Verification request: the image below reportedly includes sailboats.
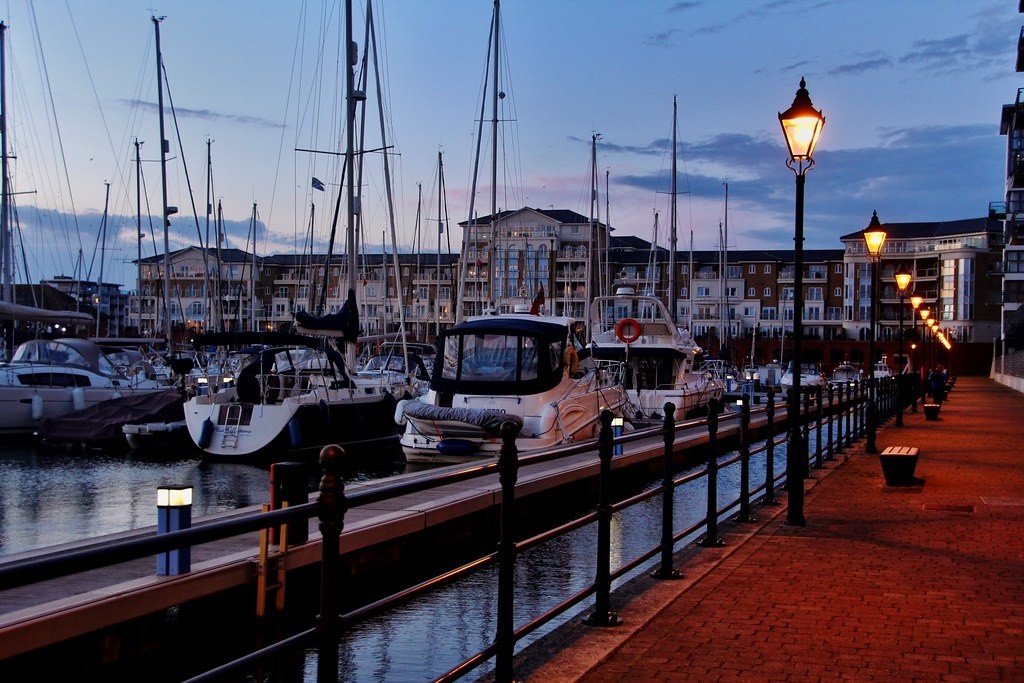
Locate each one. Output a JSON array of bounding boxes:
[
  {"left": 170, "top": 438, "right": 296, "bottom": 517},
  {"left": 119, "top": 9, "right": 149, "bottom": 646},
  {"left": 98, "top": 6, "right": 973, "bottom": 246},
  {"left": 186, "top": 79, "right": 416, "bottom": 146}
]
[{"left": 0, "top": 0, "right": 515, "bottom": 461}]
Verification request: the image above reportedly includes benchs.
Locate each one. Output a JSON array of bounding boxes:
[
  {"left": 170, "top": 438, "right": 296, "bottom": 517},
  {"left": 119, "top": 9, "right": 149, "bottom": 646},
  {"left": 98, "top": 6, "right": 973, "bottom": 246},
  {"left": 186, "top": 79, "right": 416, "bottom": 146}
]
[
  {"left": 923, "top": 404, "right": 940, "bottom": 420},
  {"left": 880, "top": 447, "right": 920, "bottom": 486},
  {"left": 943, "top": 376, "right": 956, "bottom": 401}
]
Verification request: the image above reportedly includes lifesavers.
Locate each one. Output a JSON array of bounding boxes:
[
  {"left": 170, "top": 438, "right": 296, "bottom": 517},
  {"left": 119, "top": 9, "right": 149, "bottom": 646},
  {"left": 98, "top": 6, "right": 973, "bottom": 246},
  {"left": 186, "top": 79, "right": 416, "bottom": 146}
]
[
  {"left": 563, "top": 346, "right": 579, "bottom": 374},
  {"left": 616, "top": 318, "right": 641, "bottom": 343}
]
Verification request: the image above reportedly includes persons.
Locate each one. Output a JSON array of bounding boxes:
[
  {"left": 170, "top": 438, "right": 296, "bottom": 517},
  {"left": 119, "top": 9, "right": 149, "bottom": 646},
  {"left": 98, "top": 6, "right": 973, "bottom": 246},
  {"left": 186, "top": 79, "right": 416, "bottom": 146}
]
[
  {"left": 765, "top": 378, "right": 769, "bottom": 386},
  {"left": 928, "top": 365, "right": 949, "bottom": 407}
]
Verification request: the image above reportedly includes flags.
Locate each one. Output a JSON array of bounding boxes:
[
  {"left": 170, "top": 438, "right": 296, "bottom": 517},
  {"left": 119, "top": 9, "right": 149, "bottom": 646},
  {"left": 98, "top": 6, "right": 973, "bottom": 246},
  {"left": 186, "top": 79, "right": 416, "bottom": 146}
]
[{"left": 530, "top": 288, "right": 544, "bottom": 316}]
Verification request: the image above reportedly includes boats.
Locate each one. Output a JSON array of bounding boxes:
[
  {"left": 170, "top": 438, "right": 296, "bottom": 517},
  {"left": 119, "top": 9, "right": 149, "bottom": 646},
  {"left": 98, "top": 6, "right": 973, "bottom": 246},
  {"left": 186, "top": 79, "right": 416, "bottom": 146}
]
[
  {"left": 587, "top": 293, "right": 723, "bottom": 422},
  {"left": 394, "top": 314, "right": 630, "bottom": 465},
  {"left": 868, "top": 363, "right": 893, "bottom": 387},
  {"left": 827, "top": 364, "right": 862, "bottom": 395},
  {"left": 779, "top": 357, "right": 827, "bottom": 398}
]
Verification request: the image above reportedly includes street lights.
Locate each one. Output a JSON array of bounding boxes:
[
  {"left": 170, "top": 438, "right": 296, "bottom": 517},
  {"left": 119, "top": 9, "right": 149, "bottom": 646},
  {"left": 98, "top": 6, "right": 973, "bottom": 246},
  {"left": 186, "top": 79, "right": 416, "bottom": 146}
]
[
  {"left": 893, "top": 262, "right": 912, "bottom": 428},
  {"left": 919, "top": 302, "right": 931, "bottom": 403},
  {"left": 862, "top": 210, "right": 888, "bottom": 453},
  {"left": 926, "top": 315, "right": 951, "bottom": 398},
  {"left": 909, "top": 288, "right": 923, "bottom": 412},
  {"left": 778, "top": 78, "right": 825, "bottom": 526}
]
